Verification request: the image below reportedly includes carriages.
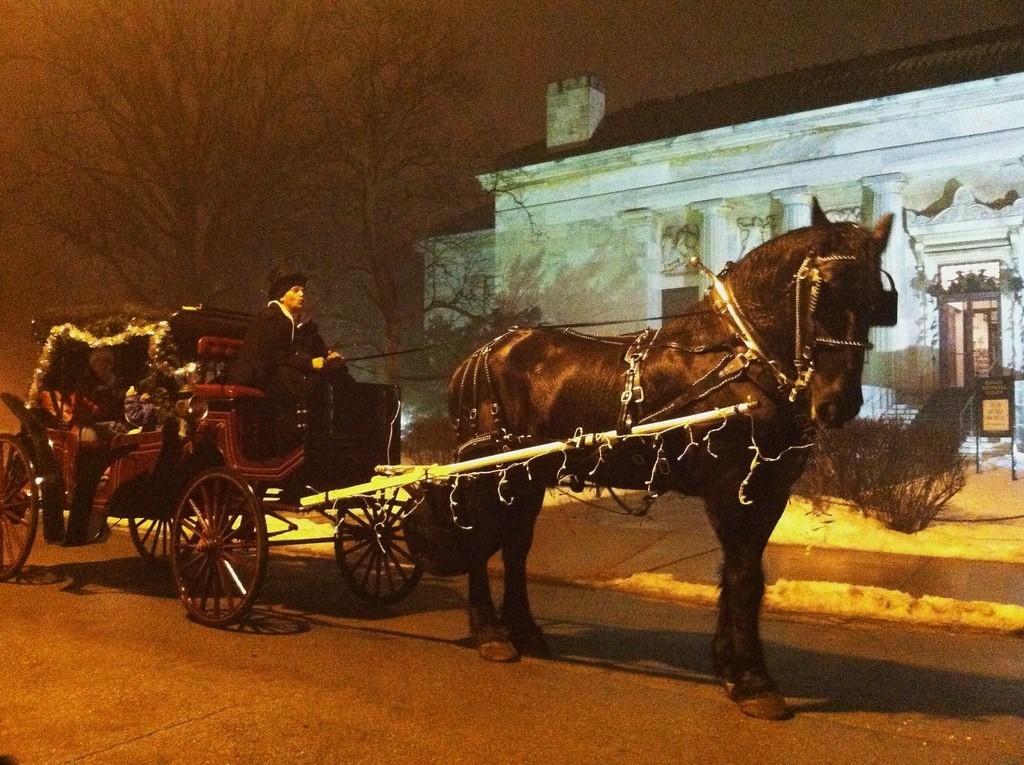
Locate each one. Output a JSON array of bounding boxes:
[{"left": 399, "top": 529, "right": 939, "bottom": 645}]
[{"left": 0, "top": 196, "right": 898, "bottom": 723}]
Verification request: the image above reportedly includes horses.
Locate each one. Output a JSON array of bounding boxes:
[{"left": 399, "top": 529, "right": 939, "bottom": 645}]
[{"left": 446, "top": 198, "right": 894, "bottom": 722}]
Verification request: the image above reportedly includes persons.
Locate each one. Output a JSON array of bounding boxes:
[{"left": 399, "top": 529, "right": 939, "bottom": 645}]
[
  {"left": 223, "top": 262, "right": 348, "bottom": 455},
  {"left": 58, "top": 347, "right": 164, "bottom": 430}
]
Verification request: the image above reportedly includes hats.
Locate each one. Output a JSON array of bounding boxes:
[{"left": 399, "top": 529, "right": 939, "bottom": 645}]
[{"left": 266, "top": 271, "right": 307, "bottom": 300}]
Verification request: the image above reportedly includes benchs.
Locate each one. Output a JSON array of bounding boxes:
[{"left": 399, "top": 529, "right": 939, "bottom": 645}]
[{"left": 193, "top": 335, "right": 269, "bottom": 409}]
